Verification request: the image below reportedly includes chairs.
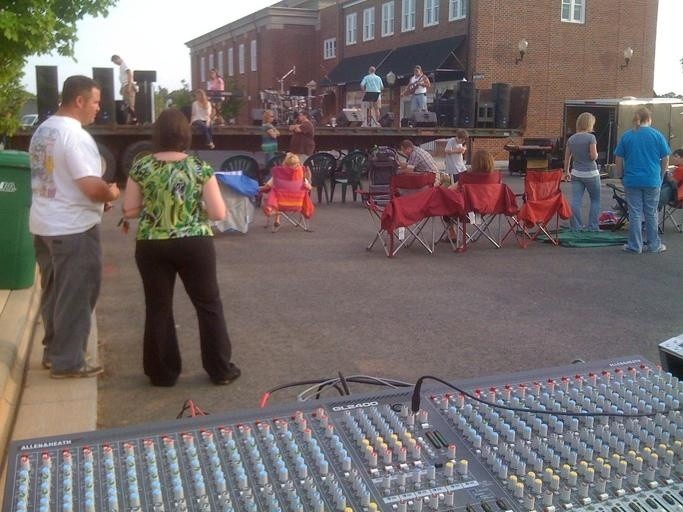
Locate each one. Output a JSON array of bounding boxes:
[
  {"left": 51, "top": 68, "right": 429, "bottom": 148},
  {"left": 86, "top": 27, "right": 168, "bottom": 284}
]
[
  {"left": 262, "top": 154, "right": 285, "bottom": 182},
  {"left": 604, "top": 174, "right": 677, "bottom": 234},
  {"left": 263, "top": 165, "right": 313, "bottom": 232},
  {"left": 657, "top": 199, "right": 682, "bottom": 232},
  {"left": 334, "top": 151, "right": 369, "bottom": 202},
  {"left": 219, "top": 155, "right": 259, "bottom": 179},
  {"left": 301, "top": 152, "right": 338, "bottom": 206},
  {"left": 354, "top": 169, "right": 572, "bottom": 258}
]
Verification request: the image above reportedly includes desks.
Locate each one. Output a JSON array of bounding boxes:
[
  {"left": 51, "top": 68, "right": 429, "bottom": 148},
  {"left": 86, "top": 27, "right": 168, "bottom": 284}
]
[
  {"left": 503, "top": 145, "right": 553, "bottom": 174},
  {"left": 207, "top": 171, "right": 259, "bottom": 234}
]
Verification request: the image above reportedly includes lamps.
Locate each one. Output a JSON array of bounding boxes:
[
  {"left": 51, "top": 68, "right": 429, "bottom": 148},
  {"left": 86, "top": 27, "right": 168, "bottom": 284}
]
[
  {"left": 619, "top": 46, "right": 633, "bottom": 70},
  {"left": 515, "top": 38, "right": 527, "bottom": 65}
]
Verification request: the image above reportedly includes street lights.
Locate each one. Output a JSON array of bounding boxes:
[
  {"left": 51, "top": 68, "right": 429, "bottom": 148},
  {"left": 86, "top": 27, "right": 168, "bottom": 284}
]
[
  {"left": 385, "top": 69, "right": 396, "bottom": 113},
  {"left": 309, "top": 79, "right": 316, "bottom": 108}
]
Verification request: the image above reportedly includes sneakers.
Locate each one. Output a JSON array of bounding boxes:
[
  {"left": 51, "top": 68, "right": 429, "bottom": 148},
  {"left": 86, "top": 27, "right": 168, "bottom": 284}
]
[
  {"left": 40, "top": 349, "right": 104, "bottom": 379},
  {"left": 210, "top": 366, "right": 241, "bottom": 385},
  {"left": 623, "top": 244, "right": 642, "bottom": 254},
  {"left": 647, "top": 244, "right": 667, "bottom": 253}
]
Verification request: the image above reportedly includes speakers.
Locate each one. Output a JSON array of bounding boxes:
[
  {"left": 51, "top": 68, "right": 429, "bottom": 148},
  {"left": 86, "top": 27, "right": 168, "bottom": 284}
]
[
  {"left": 337, "top": 108, "right": 365, "bottom": 126},
  {"left": 512, "top": 86, "right": 528, "bottom": 130},
  {"left": 94, "top": 67, "right": 114, "bottom": 126},
  {"left": 492, "top": 82, "right": 511, "bottom": 129},
  {"left": 252, "top": 109, "right": 276, "bottom": 125},
  {"left": 414, "top": 111, "right": 438, "bottom": 127},
  {"left": 457, "top": 81, "right": 476, "bottom": 128},
  {"left": 36, "top": 66, "right": 59, "bottom": 121}
]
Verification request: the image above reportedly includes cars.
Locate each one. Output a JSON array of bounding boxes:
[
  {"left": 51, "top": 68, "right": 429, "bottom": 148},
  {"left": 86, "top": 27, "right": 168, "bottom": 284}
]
[{"left": 18, "top": 112, "right": 39, "bottom": 128}]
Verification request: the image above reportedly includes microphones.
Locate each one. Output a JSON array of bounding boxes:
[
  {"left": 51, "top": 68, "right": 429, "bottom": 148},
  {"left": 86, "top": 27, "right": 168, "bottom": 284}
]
[{"left": 291, "top": 65, "right": 297, "bottom": 78}]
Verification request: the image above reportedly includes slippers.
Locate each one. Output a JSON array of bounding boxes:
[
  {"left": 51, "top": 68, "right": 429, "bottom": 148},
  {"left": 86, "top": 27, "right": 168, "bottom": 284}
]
[{"left": 440, "top": 235, "right": 457, "bottom": 243}]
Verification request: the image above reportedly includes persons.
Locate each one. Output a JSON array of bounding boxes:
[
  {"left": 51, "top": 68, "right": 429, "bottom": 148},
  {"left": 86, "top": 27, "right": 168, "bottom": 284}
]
[
  {"left": 124, "top": 107, "right": 242, "bottom": 388},
  {"left": 613, "top": 106, "right": 672, "bottom": 254},
  {"left": 398, "top": 139, "right": 438, "bottom": 188},
  {"left": 188, "top": 88, "right": 216, "bottom": 149},
  {"left": 443, "top": 127, "right": 470, "bottom": 223},
  {"left": 360, "top": 65, "right": 385, "bottom": 127},
  {"left": 27, "top": 75, "right": 122, "bottom": 379},
  {"left": 563, "top": 112, "right": 606, "bottom": 233},
  {"left": 255, "top": 109, "right": 317, "bottom": 227},
  {"left": 439, "top": 148, "right": 495, "bottom": 242},
  {"left": 111, "top": 54, "right": 139, "bottom": 125},
  {"left": 640, "top": 148, "right": 683, "bottom": 232},
  {"left": 404, "top": 64, "right": 431, "bottom": 128},
  {"left": 208, "top": 66, "right": 226, "bottom": 111}
]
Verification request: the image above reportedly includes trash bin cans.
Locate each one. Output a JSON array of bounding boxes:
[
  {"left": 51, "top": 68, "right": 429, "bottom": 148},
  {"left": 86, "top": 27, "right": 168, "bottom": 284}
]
[
  {"left": 608, "top": 164, "right": 616, "bottom": 176},
  {"left": 1, "top": 149, "right": 36, "bottom": 290}
]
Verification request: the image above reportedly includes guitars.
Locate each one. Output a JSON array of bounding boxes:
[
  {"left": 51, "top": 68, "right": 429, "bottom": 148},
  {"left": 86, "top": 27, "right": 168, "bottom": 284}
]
[{"left": 408, "top": 72, "right": 435, "bottom": 93}]
